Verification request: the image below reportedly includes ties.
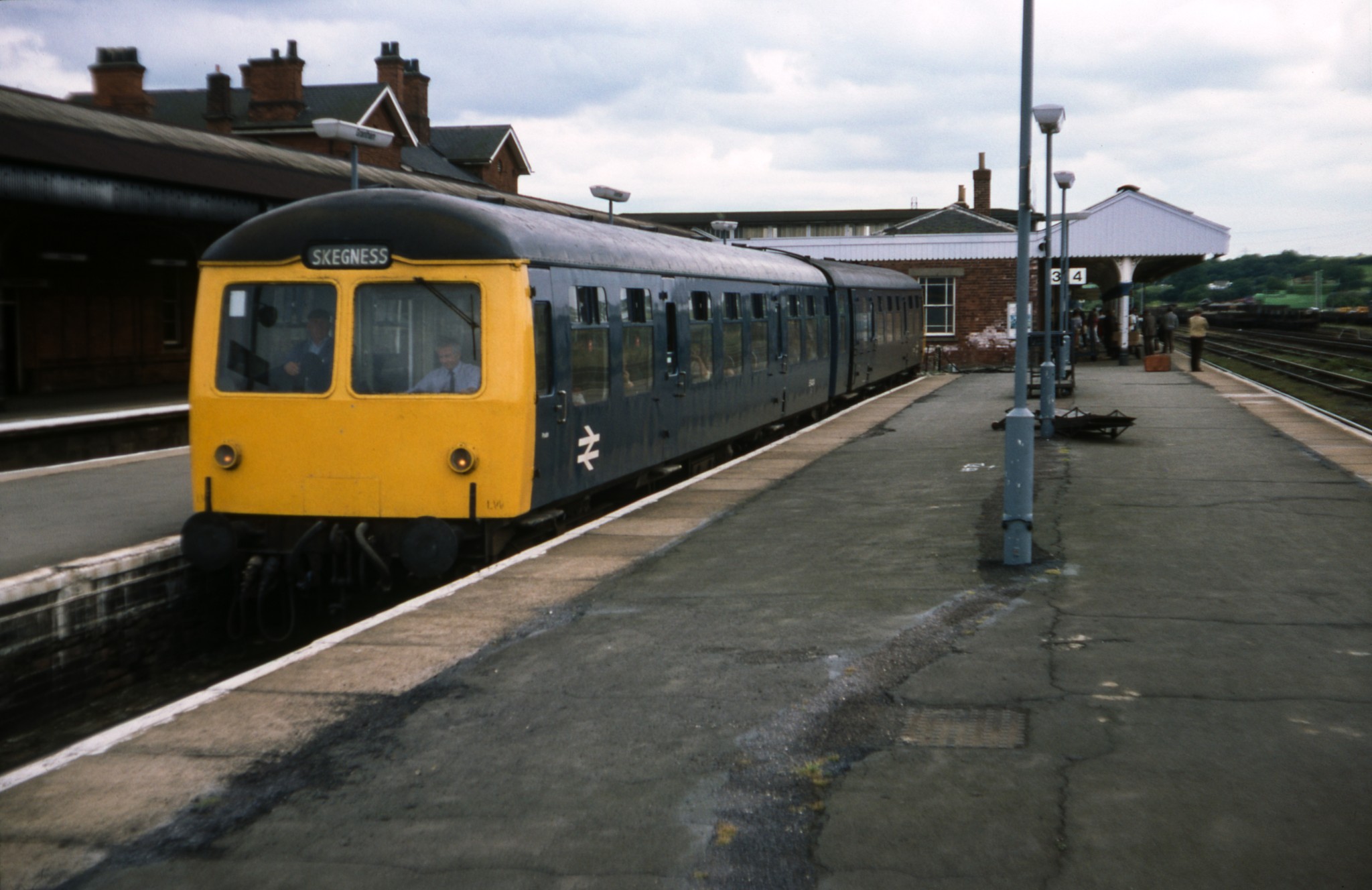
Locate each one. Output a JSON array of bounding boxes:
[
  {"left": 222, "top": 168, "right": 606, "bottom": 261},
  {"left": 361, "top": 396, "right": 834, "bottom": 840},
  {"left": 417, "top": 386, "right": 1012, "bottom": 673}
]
[{"left": 447, "top": 369, "right": 455, "bottom": 394}]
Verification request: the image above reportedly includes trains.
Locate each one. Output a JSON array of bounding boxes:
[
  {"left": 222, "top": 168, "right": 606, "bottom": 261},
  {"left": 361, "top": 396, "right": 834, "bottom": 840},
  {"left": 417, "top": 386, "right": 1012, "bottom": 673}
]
[{"left": 179, "top": 187, "right": 924, "bottom": 582}]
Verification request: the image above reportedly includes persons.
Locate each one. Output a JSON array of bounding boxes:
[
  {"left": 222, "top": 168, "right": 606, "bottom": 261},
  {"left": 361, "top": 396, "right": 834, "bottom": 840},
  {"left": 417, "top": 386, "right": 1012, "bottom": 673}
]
[
  {"left": 689, "top": 356, "right": 711, "bottom": 382},
  {"left": 572, "top": 385, "right": 586, "bottom": 407},
  {"left": 623, "top": 370, "right": 634, "bottom": 388},
  {"left": 1189, "top": 307, "right": 1209, "bottom": 372},
  {"left": 404, "top": 337, "right": 481, "bottom": 395},
  {"left": 271, "top": 308, "right": 334, "bottom": 393},
  {"left": 1070, "top": 303, "right": 1179, "bottom": 363}
]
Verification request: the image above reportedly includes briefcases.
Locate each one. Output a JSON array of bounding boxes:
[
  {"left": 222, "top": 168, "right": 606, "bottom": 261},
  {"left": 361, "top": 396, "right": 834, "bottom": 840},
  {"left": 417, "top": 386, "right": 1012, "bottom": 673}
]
[{"left": 1143, "top": 354, "right": 1171, "bottom": 372}]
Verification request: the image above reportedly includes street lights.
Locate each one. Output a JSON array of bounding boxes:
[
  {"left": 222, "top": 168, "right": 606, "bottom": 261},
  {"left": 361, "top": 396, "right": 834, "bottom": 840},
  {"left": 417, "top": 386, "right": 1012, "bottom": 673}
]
[
  {"left": 1032, "top": 100, "right": 1066, "bottom": 442},
  {"left": 311, "top": 119, "right": 394, "bottom": 192},
  {"left": 1053, "top": 169, "right": 1076, "bottom": 389},
  {"left": 710, "top": 218, "right": 737, "bottom": 240},
  {"left": 589, "top": 185, "right": 631, "bottom": 225}
]
